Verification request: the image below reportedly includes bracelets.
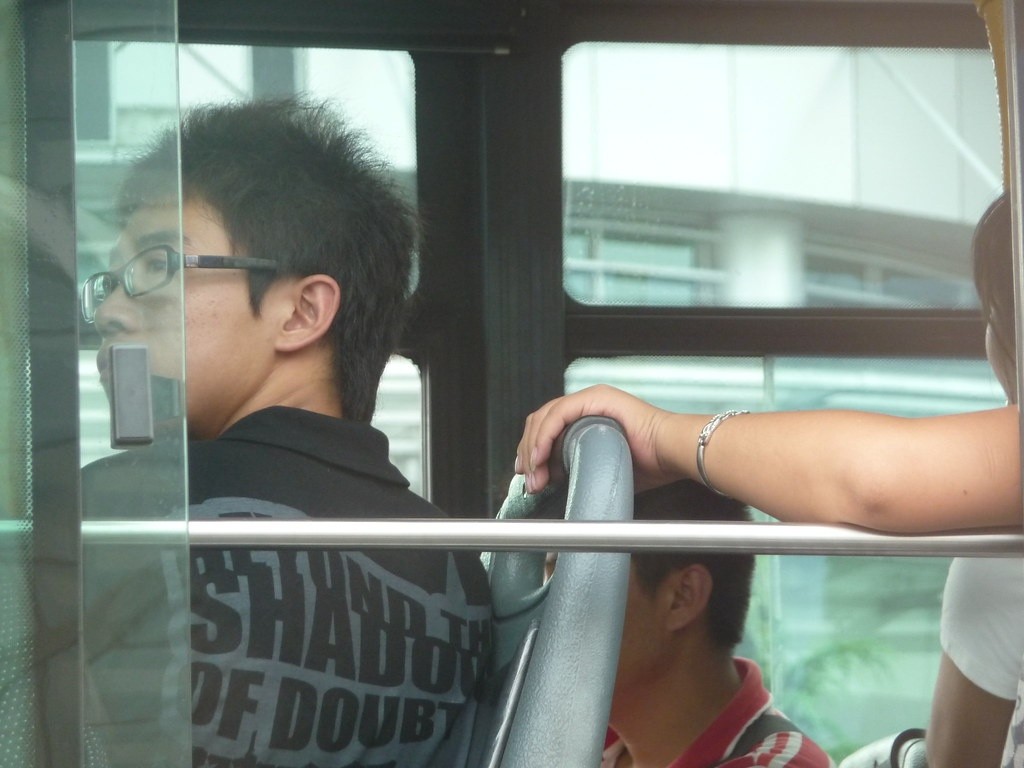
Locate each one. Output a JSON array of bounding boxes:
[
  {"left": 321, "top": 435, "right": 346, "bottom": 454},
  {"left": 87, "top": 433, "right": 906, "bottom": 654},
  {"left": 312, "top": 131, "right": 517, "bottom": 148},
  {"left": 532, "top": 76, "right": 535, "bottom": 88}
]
[{"left": 697, "top": 409, "right": 750, "bottom": 500}]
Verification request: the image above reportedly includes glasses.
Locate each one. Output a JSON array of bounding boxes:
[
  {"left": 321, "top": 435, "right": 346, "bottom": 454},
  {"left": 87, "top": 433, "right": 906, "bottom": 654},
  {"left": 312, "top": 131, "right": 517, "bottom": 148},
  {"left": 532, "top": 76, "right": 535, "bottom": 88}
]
[{"left": 81, "top": 243, "right": 287, "bottom": 323}]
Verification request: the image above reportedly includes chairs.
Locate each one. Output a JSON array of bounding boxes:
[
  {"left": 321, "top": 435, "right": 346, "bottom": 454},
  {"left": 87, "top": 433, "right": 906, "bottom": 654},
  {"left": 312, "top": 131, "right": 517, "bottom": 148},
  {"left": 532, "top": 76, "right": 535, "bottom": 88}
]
[{"left": 426, "top": 415, "right": 636, "bottom": 768}]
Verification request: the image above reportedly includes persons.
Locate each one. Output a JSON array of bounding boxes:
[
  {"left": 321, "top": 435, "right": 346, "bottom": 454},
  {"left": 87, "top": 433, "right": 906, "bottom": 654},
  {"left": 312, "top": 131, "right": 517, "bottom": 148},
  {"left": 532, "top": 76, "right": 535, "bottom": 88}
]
[
  {"left": 80, "top": 98, "right": 493, "bottom": 768},
  {"left": 514, "top": 189, "right": 1024, "bottom": 768}
]
